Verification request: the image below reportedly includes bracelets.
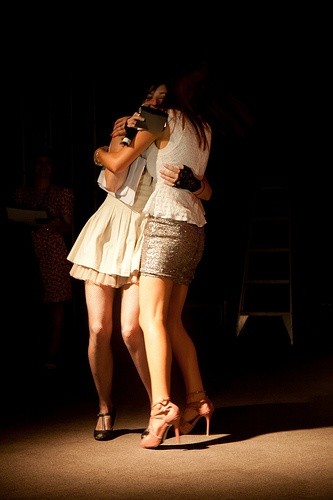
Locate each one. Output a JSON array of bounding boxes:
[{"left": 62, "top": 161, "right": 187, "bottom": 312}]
[
  {"left": 194, "top": 180, "right": 206, "bottom": 196},
  {"left": 93, "top": 150, "right": 103, "bottom": 166}
]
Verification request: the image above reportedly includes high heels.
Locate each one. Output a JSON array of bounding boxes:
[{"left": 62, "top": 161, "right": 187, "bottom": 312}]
[
  {"left": 93, "top": 411, "right": 115, "bottom": 440},
  {"left": 178, "top": 397, "right": 213, "bottom": 436},
  {"left": 140, "top": 398, "right": 181, "bottom": 449}
]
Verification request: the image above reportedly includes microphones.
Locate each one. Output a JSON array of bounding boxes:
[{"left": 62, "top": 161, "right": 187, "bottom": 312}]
[{"left": 122, "top": 102, "right": 148, "bottom": 145}]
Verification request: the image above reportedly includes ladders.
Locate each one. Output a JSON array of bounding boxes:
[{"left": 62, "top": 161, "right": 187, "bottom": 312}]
[{"left": 237, "top": 201, "right": 296, "bottom": 355}]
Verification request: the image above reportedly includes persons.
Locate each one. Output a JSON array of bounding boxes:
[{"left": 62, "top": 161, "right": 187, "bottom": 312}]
[
  {"left": 84, "top": 75, "right": 212, "bottom": 440},
  {"left": 15, "top": 151, "right": 74, "bottom": 370},
  {"left": 93, "top": 62, "right": 214, "bottom": 448}
]
